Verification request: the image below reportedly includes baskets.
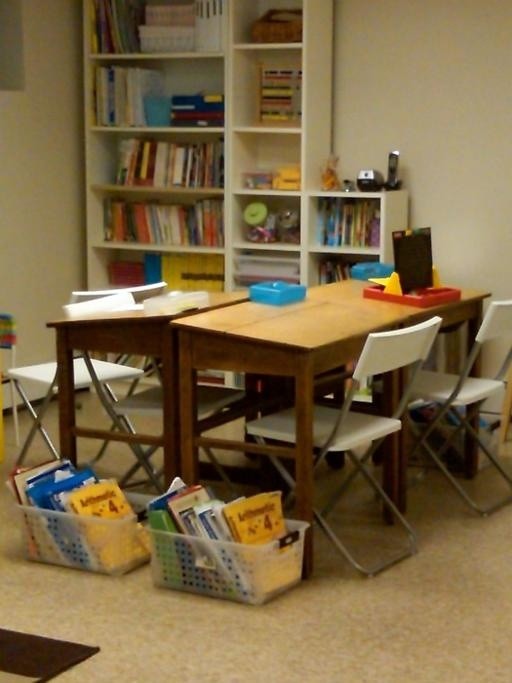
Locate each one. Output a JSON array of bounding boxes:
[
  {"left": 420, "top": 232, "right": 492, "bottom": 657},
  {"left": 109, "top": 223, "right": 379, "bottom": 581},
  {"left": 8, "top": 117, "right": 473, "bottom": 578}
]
[{"left": 14, "top": 492, "right": 162, "bottom": 578}]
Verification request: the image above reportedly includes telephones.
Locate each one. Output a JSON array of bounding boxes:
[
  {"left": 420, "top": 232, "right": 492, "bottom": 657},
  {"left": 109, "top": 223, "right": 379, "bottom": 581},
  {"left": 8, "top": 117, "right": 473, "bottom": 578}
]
[{"left": 383, "top": 151, "right": 403, "bottom": 191}]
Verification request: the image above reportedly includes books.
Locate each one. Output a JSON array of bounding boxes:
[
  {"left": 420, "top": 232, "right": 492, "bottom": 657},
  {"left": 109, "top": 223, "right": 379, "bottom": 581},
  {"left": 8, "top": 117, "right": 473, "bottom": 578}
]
[
  {"left": 91, "top": 350, "right": 226, "bottom": 387},
  {"left": 312, "top": 196, "right": 380, "bottom": 285},
  {"left": 344, "top": 362, "right": 494, "bottom": 469}
]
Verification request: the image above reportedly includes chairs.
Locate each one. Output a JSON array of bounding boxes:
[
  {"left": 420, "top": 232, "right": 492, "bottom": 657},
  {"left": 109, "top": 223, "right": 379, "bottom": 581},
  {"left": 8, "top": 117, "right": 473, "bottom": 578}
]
[{"left": 0, "top": 289, "right": 512, "bottom": 577}]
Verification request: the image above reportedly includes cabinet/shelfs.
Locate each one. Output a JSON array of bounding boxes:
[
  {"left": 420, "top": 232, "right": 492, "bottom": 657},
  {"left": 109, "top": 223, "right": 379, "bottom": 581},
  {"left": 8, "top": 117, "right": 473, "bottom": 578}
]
[{"left": 79, "top": 0, "right": 411, "bottom": 397}]
